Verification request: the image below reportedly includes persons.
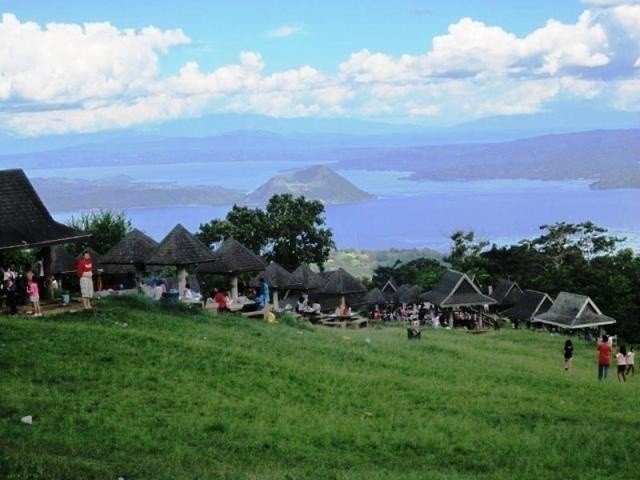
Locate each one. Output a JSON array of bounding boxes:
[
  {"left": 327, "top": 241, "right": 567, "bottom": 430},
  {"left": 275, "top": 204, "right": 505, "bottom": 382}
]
[
  {"left": 597, "top": 335, "right": 635, "bottom": 383},
  {"left": 137, "top": 277, "right": 270, "bottom": 313},
  {"left": 371, "top": 301, "right": 499, "bottom": 331},
  {"left": 296, "top": 296, "right": 351, "bottom": 321},
  {"left": 76, "top": 251, "right": 94, "bottom": 309},
  {"left": 563, "top": 339, "right": 574, "bottom": 371},
  {"left": 0, "top": 252, "right": 58, "bottom": 318}
]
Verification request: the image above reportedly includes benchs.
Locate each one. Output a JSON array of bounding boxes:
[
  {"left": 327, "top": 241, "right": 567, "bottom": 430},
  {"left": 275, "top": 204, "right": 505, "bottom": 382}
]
[{"left": 205, "top": 298, "right": 368, "bottom": 330}]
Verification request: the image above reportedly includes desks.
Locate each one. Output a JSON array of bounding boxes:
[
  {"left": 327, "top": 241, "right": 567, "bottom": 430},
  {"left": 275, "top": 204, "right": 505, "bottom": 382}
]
[{"left": 183, "top": 300, "right": 204, "bottom": 312}]
